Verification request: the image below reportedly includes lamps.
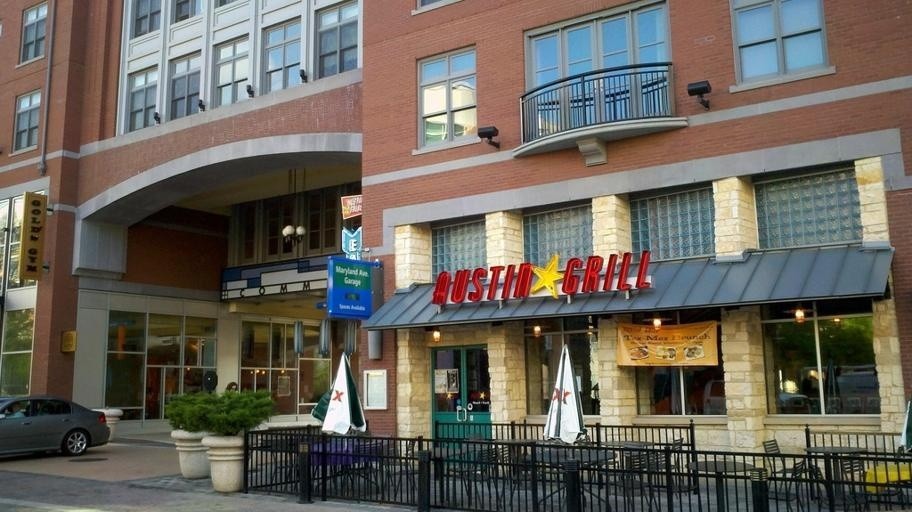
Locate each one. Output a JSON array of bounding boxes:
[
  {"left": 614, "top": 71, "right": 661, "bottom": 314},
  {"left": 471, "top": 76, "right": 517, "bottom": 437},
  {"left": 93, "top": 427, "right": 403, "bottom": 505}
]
[
  {"left": 246, "top": 85, "right": 253, "bottom": 98},
  {"left": 299, "top": 69, "right": 307, "bottom": 83},
  {"left": 475, "top": 126, "right": 500, "bottom": 150},
  {"left": 151, "top": 112, "right": 160, "bottom": 124},
  {"left": 197, "top": 100, "right": 204, "bottom": 112},
  {"left": 686, "top": 80, "right": 713, "bottom": 112},
  {"left": 280, "top": 168, "right": 308, "bottom": 247}
]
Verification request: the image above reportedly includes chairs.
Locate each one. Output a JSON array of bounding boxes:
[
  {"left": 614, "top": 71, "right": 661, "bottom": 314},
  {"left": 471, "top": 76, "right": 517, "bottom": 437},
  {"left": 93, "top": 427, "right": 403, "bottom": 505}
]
[
  {"left": 359, "top": 436, "right": 687, "bottom": 511},
  {"left": 840, "top": 455, "right": 904, "bottom": 511},
  {"left": 760, "top": 438, "right": 806, "bottom": 498}
]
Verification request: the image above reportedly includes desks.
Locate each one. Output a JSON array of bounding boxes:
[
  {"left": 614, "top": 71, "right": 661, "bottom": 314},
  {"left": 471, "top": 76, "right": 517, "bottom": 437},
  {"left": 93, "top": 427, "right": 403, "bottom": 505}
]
[
  {"left": 310, "top": 440, "right": 386, "bottom": 499},
  {"left": 801, "top": 445, "right": 868, "bottom": 508},
  {"left": 684, "top": 459, "right": 754, "bottom": 511}
]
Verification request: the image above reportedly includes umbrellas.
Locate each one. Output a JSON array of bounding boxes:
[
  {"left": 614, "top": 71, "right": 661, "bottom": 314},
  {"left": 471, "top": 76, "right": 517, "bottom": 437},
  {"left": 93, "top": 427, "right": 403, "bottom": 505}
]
[
  {"left": 899, "top": 399, "right": 912, "bottom": 449},
  {"left": 542, "top": 343, "right": 588, "bottom": 459},
  {"left": 308, "top": 351, "right": 368, "bottom": 435}
]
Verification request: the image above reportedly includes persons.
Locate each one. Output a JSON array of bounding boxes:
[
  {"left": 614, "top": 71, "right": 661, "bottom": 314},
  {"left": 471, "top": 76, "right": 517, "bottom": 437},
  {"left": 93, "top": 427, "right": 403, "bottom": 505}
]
[{"left": 6, "top": 401, "right": 26, "bottom": 419}]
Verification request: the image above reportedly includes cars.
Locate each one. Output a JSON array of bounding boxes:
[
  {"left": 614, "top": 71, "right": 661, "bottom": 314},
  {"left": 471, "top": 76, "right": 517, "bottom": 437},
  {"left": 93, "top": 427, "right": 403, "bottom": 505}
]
[{"left": 0, "top": 395, "right": 110, "bottom": 457}]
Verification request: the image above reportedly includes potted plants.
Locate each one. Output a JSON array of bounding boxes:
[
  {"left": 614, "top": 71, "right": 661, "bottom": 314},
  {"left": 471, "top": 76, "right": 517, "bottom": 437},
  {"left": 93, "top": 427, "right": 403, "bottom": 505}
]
[{"left": 169, "top": 387, "right": 276, "bottom": 492}]
